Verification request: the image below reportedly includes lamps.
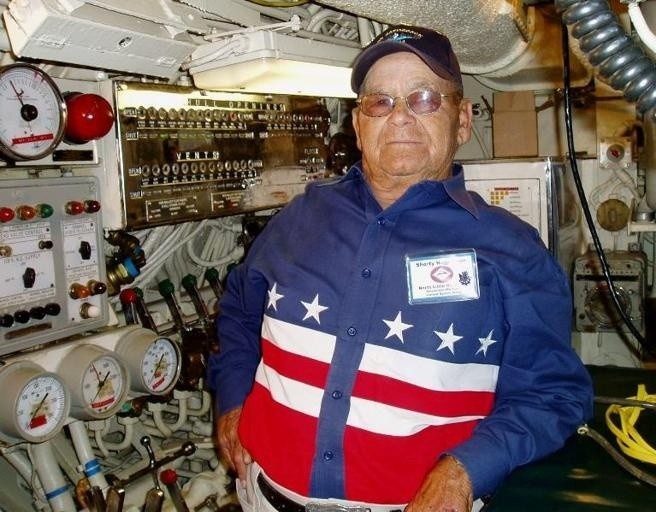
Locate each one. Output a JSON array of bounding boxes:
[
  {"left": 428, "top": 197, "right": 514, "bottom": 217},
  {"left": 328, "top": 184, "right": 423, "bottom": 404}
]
[{"left": 181, "top": 29, "right": 361, "bottom": 99}]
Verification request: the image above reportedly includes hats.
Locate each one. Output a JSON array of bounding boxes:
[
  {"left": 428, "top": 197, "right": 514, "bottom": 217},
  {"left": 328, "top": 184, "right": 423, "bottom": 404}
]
[{"left": 351, "top": 25, "right": 461, "bottom": 93}]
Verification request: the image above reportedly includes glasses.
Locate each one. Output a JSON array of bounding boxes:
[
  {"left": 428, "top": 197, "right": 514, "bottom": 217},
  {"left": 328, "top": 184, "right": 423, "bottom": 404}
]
[{"left": 356, "top": 89, "right": 456, "bottom": 117}]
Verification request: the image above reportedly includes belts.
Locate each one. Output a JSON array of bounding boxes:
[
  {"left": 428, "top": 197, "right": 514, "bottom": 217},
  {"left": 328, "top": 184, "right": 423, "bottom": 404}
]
[{"left": 257, "top": 470, "right": 380, "bottom": 512}]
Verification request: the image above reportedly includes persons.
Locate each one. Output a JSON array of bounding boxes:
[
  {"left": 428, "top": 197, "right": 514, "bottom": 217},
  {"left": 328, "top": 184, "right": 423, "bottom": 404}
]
[{"left": 202, "top": 23, "right": 595, "bottom": 512}]
